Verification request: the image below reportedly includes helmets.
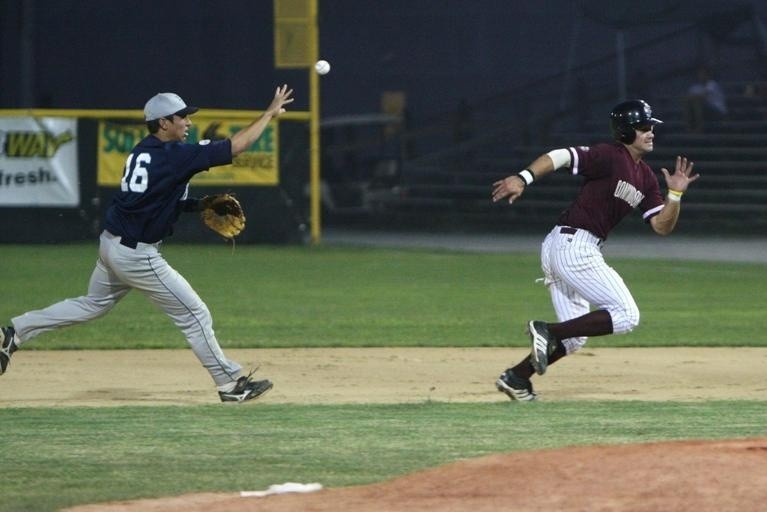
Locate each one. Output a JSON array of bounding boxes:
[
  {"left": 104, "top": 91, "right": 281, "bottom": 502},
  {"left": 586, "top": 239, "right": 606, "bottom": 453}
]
[{"left": 609, "top": 98, "right": 664, "bottom": 129}]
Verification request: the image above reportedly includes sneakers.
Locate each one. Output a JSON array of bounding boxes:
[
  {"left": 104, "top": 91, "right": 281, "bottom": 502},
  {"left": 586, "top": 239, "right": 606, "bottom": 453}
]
[
  {"left": 493, "top": 368, "right": 536, "bottom": 401},
  {"left": 526, "top": 318, "right": 558, "bottom": 376},
  {"left": 0, "top": 326, "right": 19, "bottom": 376},
  {"left": 216, "top": 374, "right": 273, "bottom": 403}
]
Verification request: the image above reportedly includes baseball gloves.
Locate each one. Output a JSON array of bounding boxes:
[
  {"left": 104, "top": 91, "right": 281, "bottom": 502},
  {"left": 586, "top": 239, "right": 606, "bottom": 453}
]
[{"left": 199, "top": 192, "right": 248, "bottom": 239}]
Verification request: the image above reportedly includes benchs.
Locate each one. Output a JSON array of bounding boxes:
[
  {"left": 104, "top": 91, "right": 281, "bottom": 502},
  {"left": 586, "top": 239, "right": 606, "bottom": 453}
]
[{"left": 394, "top": 35, "right": 767, "bottom": 237}]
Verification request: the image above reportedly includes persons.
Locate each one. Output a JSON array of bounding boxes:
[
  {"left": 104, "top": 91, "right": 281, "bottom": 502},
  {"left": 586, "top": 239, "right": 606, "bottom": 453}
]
[
  {"left": 0, "top": 82, "right": 298, "bottom": 402},
  {"left": 487, "top": 97, "right": 703, "bottom": 402}
]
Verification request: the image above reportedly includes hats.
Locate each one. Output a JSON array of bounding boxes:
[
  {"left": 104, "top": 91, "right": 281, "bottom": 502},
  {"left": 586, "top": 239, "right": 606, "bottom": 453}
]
[{"left": 143, "top": 92, "right": 198, "bottom": 123}]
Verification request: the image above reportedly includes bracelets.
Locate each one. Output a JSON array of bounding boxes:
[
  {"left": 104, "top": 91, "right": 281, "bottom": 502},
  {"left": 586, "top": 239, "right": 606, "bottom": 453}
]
[
  {"left": 516, "top": 169, "right": 535, "bottom": 188},
  {"left": 667, "top": 189, "right": 682, "bottom": 196},
  {"left": 667, "top": 193, "right": 682, "bottom": 202}
]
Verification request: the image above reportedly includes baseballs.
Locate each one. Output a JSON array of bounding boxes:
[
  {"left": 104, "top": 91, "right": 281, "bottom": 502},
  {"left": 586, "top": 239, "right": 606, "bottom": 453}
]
[{"left": 316, "top": 60, "right": 331, "bottom": 75}]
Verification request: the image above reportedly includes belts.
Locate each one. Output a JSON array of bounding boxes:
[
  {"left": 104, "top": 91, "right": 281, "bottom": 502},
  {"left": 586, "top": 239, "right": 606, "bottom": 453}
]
[
  {"left": 553, "top": 225, "right": 601, "bottom": 246},
  {"left": 101, "top": 229, "right": 153, "bottom": 250}
]
